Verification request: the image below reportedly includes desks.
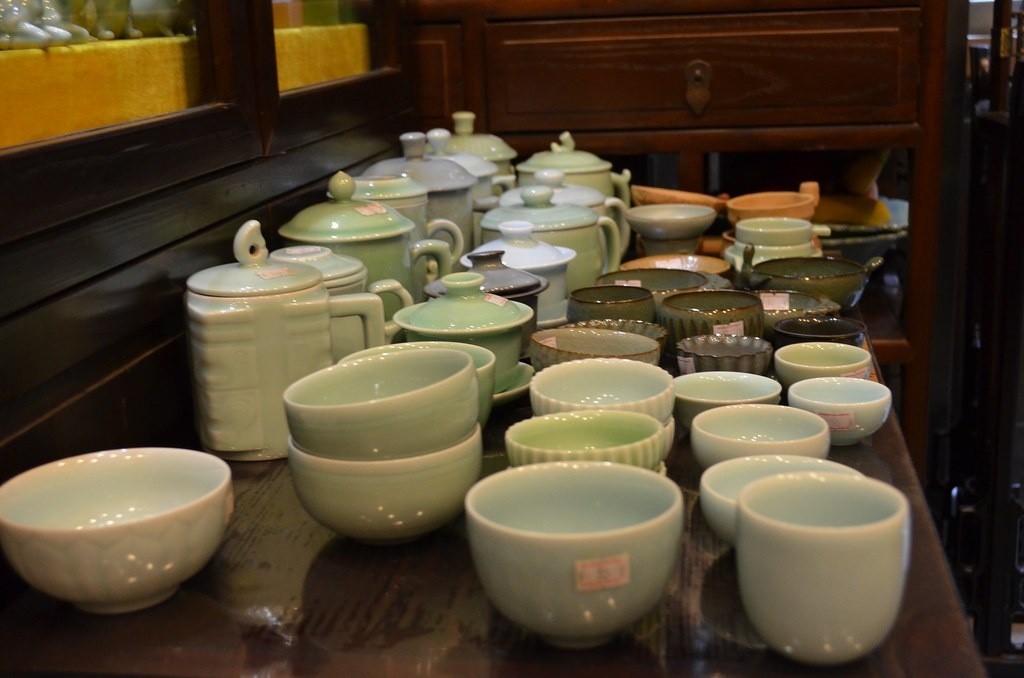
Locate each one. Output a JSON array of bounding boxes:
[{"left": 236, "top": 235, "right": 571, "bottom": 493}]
[{"left": 0, "top": 306, "right": 988, "bottom": 678}]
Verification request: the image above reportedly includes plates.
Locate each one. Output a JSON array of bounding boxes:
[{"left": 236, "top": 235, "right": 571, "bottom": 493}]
[
  {"left": 538, "top": 307, "right": 572, "bottom": 329},
  {"left": 492, "top": 359, "right": 536, "bottom": 402}
]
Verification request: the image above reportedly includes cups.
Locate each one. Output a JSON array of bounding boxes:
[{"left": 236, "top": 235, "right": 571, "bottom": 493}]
[
  {"left": 737, "top": 471, "right": 911, "bottom": 662},
  {"left": 182, "top": 110, "right": 631, "bottom": 461}
]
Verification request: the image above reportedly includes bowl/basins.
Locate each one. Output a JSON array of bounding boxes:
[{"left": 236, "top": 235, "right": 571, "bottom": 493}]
[
  {"left": 465, "top": 319, "right": 685, "bottom": 648},
  {"left": 772, "top": 341, "right": 891, "bottom": 447},
  {"left": 283, "top": 341, "right": 496, "bottom": 539},
  {"left": 660, "top": 288, "right": 865, "bottom": 547},
  {"left": 724, "top": 182, "right": 868, "bottom": 348},
  {"left": 0, "top": 448, "right": 233, "bottom": 614},
  {"left": 591, "top": 183, "right": 733, "bottom": 322}
]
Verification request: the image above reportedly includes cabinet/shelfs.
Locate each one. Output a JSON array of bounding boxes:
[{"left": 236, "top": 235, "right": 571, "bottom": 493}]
[{"left": 410, "top": 0, "right": 947, "bottom": 482}]
